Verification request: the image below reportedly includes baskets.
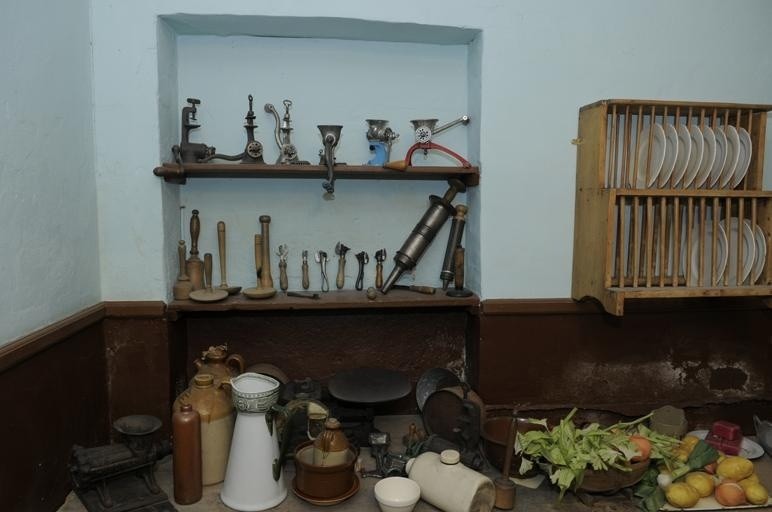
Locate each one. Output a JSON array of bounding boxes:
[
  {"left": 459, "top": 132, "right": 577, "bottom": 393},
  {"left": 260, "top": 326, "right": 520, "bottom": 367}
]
[{"left": 579, "top": 460, "right": 651, "bottom": 492}]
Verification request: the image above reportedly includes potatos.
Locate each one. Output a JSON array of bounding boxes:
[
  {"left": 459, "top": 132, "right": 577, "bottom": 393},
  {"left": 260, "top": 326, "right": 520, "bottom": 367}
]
[{"left": 663, "top": 436, "right": 768, "bottom": 508}]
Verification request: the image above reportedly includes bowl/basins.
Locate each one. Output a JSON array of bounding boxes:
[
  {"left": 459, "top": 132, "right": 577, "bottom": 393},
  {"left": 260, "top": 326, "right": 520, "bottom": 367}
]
[
  {"left": 415, "top": 365, "right": 468, "bottom": 415},
  {"left": 484, "top": 406, "right": 658, "bottom": 495},
  {"left": 290, "top": 440, "right": 363, "bottom": 501},
  {"left": 372, "top": 476, "right": 422, "bottom": 509}
]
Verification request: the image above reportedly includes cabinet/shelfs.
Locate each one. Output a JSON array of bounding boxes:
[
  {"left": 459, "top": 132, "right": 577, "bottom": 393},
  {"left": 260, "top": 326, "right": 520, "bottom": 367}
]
[
  {"left": 163, "top": 162, "right": 481, "bottom": 313},
  {"left": 571, "top": 98, "right": 772, "bottom": 318}
]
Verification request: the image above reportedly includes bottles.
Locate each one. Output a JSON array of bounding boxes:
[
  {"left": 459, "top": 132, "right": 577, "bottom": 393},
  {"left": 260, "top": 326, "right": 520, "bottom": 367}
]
[
  {"left": 313, "top": 418, "right": 350, "bottom": 467},
  {"left": 172, "top": 403, "right": 203, "bottom": 504}
]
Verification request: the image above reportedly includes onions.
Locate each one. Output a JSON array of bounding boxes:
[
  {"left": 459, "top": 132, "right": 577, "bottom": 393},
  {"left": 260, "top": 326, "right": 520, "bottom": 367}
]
[{"left": 628, "top": 436, "right": 651, "bottom": 462}]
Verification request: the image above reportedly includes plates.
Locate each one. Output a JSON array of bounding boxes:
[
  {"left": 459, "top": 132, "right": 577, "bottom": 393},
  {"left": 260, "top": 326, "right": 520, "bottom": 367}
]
[
  {"left": 685, "top": 429, "right": 765, "bottom": 460},
  {"left": 633, "top": 118, "right": 767, "bottom": 289}
]
